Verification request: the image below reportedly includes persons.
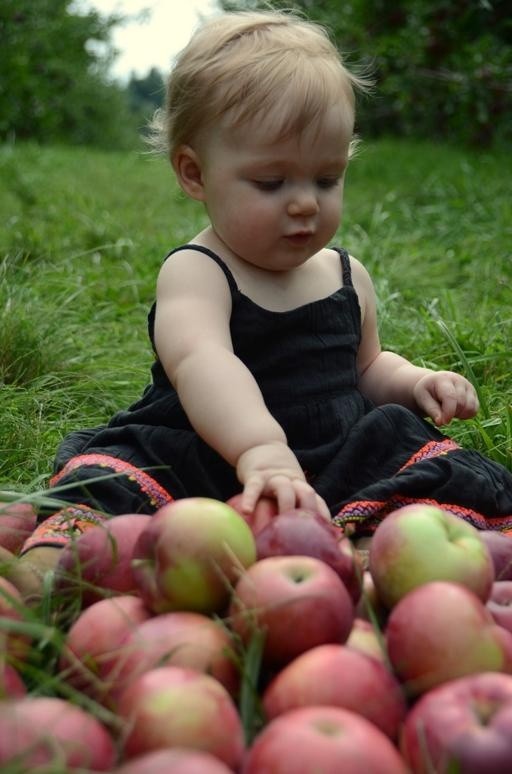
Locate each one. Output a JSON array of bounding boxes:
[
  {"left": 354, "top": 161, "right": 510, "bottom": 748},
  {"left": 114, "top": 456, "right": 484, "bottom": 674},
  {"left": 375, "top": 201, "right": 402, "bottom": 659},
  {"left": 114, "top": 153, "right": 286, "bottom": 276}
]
[{"left": 17, "top": 7, "right": 512, "bottom": 560}]
